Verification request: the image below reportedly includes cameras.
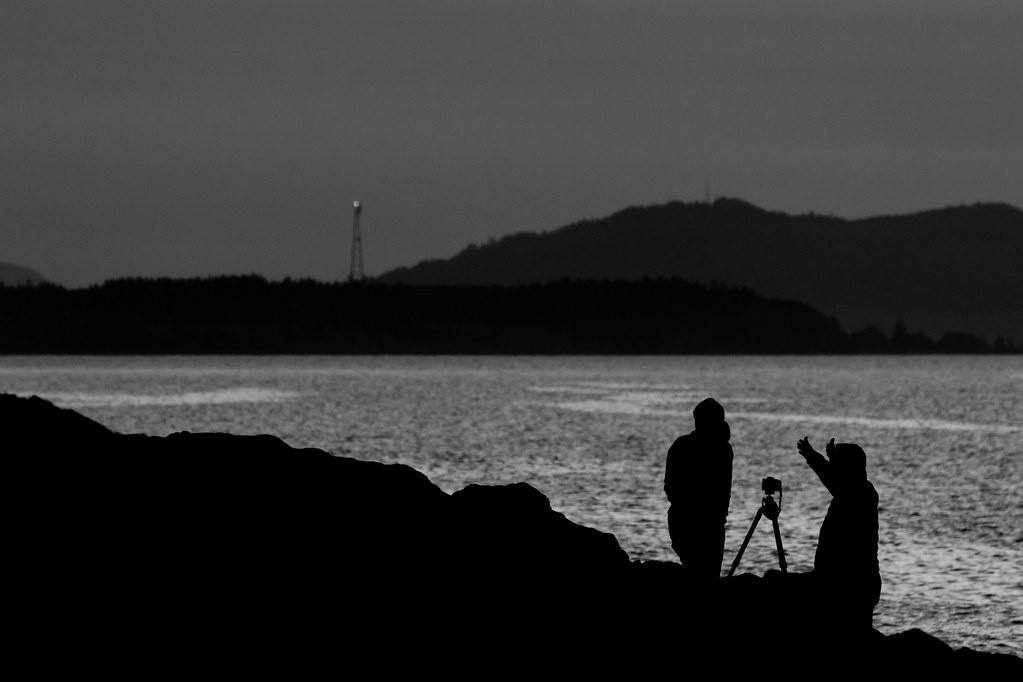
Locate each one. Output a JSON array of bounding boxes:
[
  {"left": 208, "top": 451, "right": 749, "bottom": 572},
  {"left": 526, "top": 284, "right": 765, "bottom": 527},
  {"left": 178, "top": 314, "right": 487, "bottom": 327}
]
[{"left": 762, "top": 476, "right": 782, "bottom": 495}]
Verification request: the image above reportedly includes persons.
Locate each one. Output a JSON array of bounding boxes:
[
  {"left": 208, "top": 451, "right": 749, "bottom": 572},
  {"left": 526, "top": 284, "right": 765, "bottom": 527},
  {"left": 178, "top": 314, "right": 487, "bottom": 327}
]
[
  {"left": 796, "top": 432, "right": 883, "bottom": 623},
  {"left": 661, "top": 396, "right": 733, "bottom": 582}
]
